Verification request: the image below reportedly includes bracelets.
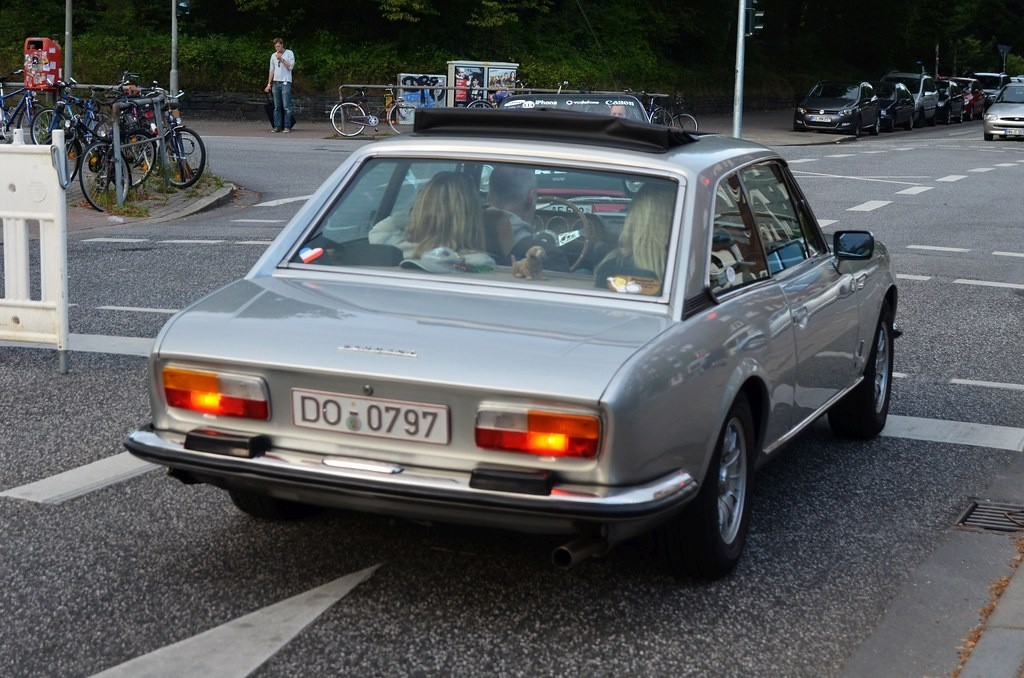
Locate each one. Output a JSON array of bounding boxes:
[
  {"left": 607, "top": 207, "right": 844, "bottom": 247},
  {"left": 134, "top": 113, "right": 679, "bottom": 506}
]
[
  {"left": 267, "top": 84, "right": 272, "bottom": 86},
  {"left": 281, "top": 59, "right": 285, "bottom": 63}
]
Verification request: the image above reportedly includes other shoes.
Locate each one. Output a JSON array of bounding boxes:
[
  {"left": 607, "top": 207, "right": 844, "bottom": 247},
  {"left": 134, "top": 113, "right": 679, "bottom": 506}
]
[
  {"left": 282, "top": 127, "right": 291, "bottom": 133},
  {"left": 271, "top": 127, "right": 280, "bottom": 133}
]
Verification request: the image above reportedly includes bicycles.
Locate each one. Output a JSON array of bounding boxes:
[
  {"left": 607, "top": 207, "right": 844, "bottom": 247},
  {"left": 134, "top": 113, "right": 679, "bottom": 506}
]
[
  {"left": 329, "top": 82, "right": 416, "bottom": 137},
  {"left": 0, "top": 69, "right": 51, "bottom": 145},
  {"left": 466, "top": 78, "right": 532, "bottom": 108},
  {"left": 29, "top": 70, "right": 206, "bottom": 214},
  {"left": 625, "top": 88, "right": 699, "bottom": 132}
]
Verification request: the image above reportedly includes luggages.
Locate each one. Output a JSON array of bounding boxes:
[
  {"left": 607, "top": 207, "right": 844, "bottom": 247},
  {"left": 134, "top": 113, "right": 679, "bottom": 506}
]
[{"left": 264, "top": 91, "right": 296, "bottom": 130}]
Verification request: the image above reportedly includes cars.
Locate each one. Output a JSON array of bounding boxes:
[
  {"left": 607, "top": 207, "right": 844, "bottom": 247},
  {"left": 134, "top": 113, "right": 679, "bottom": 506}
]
[
  {"left": 941, "top": 75, "right": 987, "bottom": 122},
  {"left": 930, "top": 79, "right": 965, "bottom": 125},
  {"left": 862, "top": 80, "right": 916, "bottom": 135},
  {"left": 983, "top": 82, "right": 1024, "bottom": 141},
  {"left": 495, "top": 90, "right": 677, "bottom": 126},
  {"left": 793, "top": 79, "right": 883, "bottom": 138}
]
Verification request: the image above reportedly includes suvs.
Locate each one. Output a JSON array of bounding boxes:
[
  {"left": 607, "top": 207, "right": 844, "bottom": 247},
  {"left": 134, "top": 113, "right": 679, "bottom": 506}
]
[
  {"left": 968, "top": 72, "right": 1024, "bottom": 112},
  {"left": 880, "top": 70, "right": 940, "bottom": 129}
]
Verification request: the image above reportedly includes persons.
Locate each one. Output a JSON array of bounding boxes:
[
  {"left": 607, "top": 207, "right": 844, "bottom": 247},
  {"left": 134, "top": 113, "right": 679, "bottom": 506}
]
[
  {"left": 486, "top": 164, "right": 570, "bottom": 273},
  {"left": 265, "top": 38, "right": 295, "bottom": 133},
  {"left": 595, "top": 181, "right": 678, "bottom": 274},
  {"left": 611, "top": 105, "right": 630, "bottom": 119},
  {"left": 368, "top": 171, "right": 496, "bottom": 264},
  {"left": 489, "top": 72, "right": 516, "bottom": 93},
  {"left": 470, "top": 77, "right": 482, "bottom": 100}
]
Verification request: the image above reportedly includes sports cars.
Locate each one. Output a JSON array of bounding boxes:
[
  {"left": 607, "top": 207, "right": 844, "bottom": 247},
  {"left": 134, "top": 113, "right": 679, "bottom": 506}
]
[{"left": 122, "top": 103, "right": 906, "bottom": 586}]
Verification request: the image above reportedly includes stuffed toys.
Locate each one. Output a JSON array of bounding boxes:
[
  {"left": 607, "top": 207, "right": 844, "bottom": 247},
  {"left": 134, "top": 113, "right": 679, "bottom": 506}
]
[{"left": 511, "top": 246, "right": 547, "bottom": 280}]
